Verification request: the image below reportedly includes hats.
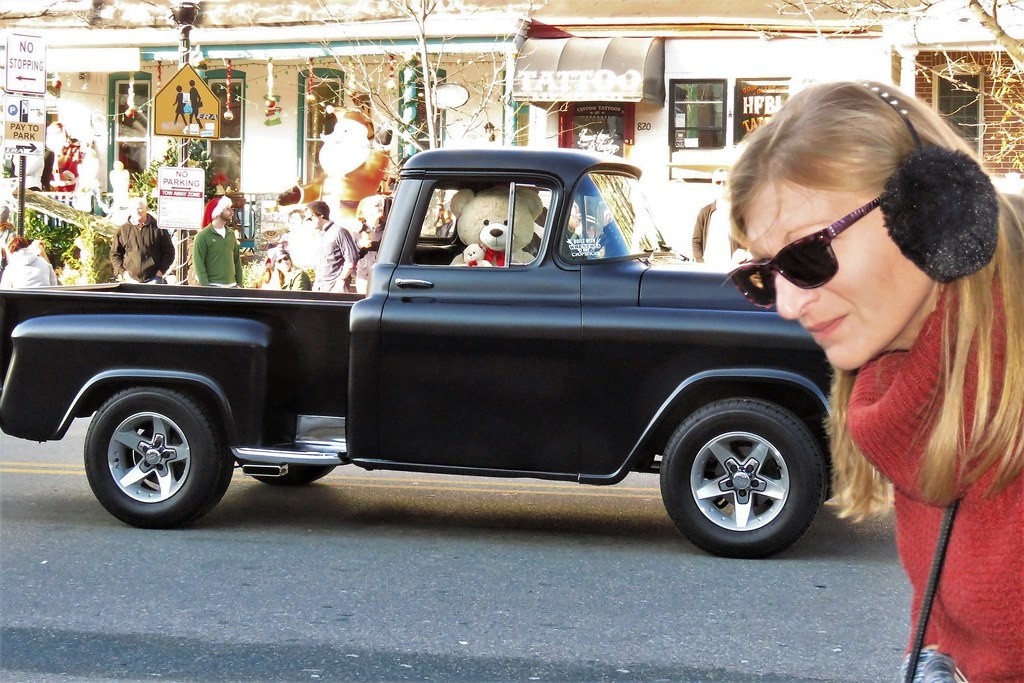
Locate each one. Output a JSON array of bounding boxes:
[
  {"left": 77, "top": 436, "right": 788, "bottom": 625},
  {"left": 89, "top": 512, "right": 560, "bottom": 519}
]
[
  {"left": 272, "top": 250, "right": 289, "bottom": 264},
  {"left": 201, "top": 194, "right": 231, "bottom": 230}
]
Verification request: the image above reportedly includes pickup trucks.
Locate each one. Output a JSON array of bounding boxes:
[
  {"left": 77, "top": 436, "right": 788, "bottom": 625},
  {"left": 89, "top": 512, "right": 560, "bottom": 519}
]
[{"left": 2, "top": 143, "right": 860, "bottom": 561}]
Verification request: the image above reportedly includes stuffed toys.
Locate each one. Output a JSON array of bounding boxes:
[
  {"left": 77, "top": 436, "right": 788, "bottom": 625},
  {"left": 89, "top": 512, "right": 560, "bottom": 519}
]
[{"left": 451, "top": 187, "right": 543, "bottom": 267}]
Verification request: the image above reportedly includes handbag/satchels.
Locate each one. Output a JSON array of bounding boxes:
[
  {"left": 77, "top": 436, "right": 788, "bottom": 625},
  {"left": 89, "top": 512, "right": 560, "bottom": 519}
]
[{"left": 901, "top": 647, "right": 956, "bottom": 683}]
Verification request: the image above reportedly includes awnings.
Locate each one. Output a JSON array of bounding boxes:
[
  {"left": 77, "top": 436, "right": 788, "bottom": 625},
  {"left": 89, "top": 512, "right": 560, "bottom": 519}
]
[{"left": 510, "top": 37, "right": 666, "bottom": 107}]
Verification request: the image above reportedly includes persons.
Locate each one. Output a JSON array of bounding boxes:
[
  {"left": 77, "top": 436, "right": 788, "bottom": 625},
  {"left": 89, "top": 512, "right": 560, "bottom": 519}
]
[
  {"left": 194, "top": 195, "right": 244, "bottom": 289},
  {"left": 728, "top": 80, "right": 1023, "bottom": 683},
  {"left": 353, "top": 196, "right": 384, "bottom": 294},
  {"left": 0, "top": 224, "right": 56, "bottom": 290},
  {"left": 305, "top": 201, "right": 358, "bottom": 293},
  {"left": 692, "top": 169, "right": 751, "bottom": 266},
  {"left": 267, "top": 249, "right": 312, "bottom": 291},
  {"left": 110, "top": 197, "right": 175, "bottom": 284},
  {"left": 11, "top": 121, "right": 80, "bottom": 193}
]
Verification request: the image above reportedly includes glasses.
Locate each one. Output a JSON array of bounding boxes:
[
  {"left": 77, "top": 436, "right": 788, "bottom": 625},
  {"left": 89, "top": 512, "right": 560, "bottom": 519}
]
[
  {"left": 715, "top": 180, "right": 725, "bottom": 185},
  {"left": 276, "top": 256, "right": 290, "bottom": 263},
  {"left": 726, "top": 193, "right": 883, "bottom": 309}
]
